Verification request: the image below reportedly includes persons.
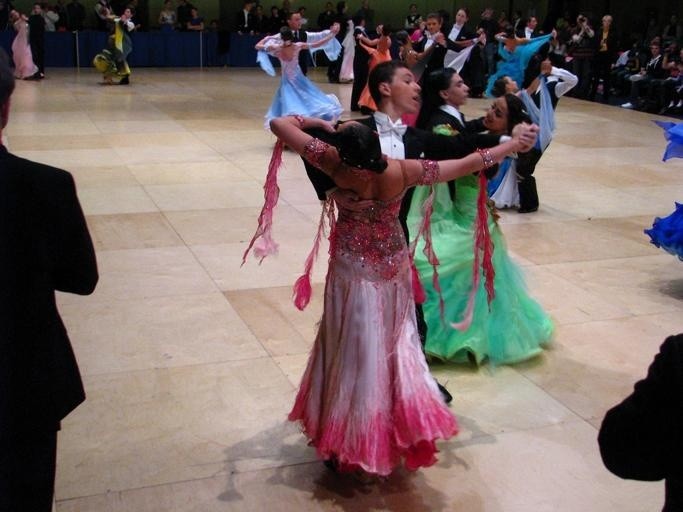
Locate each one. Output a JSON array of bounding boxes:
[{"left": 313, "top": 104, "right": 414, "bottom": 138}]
[
  {"left": 597, "top": 332, "right": 683, "bottom": 512},
  {"left": 242, "top": 62, "right": 554, "bottom": 482},
  {"left": 0, "top": 38, "right": 101, "bottom": 511},
  {"left": 257, "top": 0, "right": 391, "bottom": 147},
  {"left": 394, "top": 0, "right": 568, "bottom": 99},
  {"left": 492, "top": 53, "right": 578, "bottom": 213},
  {"left": 0, "top": 0, "right": 86, "bottom": 80},
  {"left": 93, "top": 0, "right": 150, "bottom": 84},
  {"left": 158, "top": 1, "right": 266, "bottom": 49},
  {"left": 569, "top": 14, "right": 683, "bottom": 118}
]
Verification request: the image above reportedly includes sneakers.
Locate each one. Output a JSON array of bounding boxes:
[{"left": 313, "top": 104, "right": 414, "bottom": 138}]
[{"left": 621, "top": 102, "right": 637, "bottom": 109}]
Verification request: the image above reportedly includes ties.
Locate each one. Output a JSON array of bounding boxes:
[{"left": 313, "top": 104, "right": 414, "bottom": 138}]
[{"left": 294, "top": 32, "right": 298, "bottom": 42}]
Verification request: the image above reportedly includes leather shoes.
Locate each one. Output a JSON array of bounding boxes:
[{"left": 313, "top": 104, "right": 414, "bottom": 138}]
[
  {"left": 519, "top": 207, "right": 537, "bottom": 213},
  {"left": 437, "top": 382, "right": 452, "bottom": 403},
  {"left": 120, "top": 79, "right": 129, "bottom": 85}
]
